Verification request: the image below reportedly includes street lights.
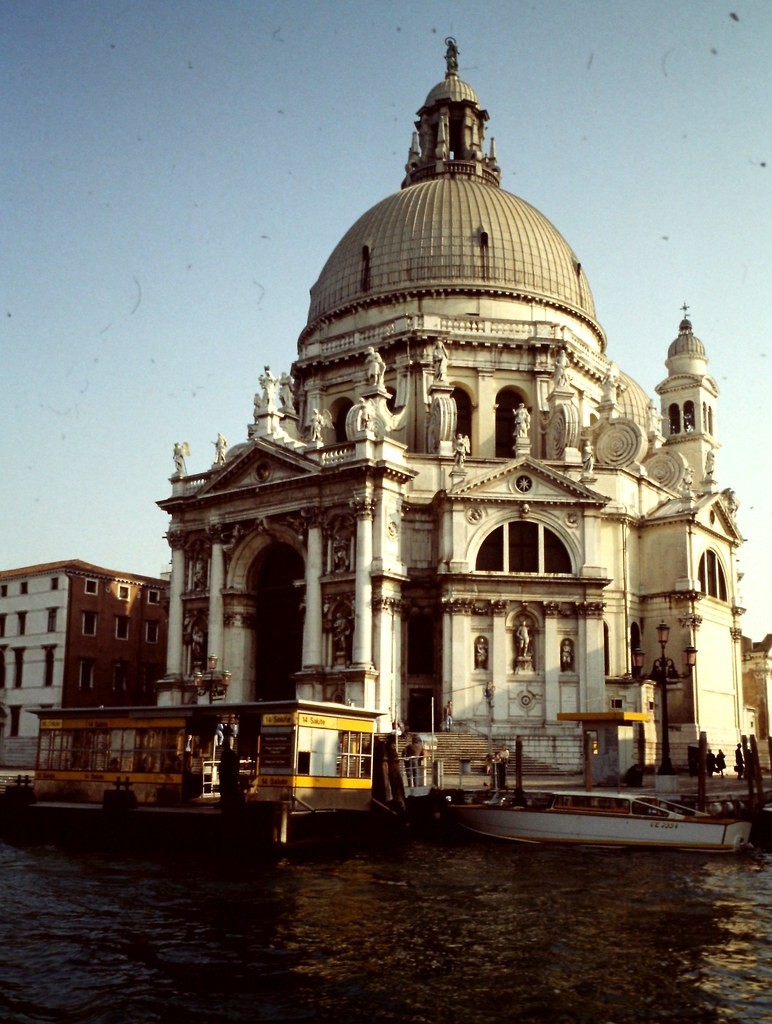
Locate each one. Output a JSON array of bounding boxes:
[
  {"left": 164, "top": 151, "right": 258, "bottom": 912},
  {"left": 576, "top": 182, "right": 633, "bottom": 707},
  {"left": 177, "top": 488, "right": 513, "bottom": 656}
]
[
  {"left": 632, "top": 620, "right": 698, "bottom": 775},
  {"left": 193, "top": 654, "right": 233, "bottom": 705}
]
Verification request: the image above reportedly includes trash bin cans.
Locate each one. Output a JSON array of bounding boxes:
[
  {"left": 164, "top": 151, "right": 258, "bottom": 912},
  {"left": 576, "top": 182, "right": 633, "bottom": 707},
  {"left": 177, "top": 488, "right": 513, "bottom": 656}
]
[{"left": 458, "top": 757, "right": 473, "bottom": 775}]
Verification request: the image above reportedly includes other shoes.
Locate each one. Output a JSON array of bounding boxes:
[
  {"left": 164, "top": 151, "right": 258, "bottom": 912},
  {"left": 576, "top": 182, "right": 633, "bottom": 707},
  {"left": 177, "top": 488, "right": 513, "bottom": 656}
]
[
  {"left": 721, "top": 775, "right": 724, "bottom": 779},
  {"left": 737, "top": 775, "right": 744, "bottom": 780},
  {"left": 709, "top": 774, "right": 712, "bottom": 777},
  {"left": 715, "top": 770, "right": 719, "bottom": 773}
]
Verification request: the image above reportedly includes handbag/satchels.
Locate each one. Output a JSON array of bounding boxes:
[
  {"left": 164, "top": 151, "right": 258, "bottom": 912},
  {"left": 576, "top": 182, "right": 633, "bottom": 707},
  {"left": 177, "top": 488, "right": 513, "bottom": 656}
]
[{"left": 734, "top": 766, "right": 739, "bottom": 771}]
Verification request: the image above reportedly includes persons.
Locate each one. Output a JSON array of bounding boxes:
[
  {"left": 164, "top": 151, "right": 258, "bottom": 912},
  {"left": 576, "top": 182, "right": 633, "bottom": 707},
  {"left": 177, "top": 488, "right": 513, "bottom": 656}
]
[
  {"left": 253, "top": 365, "right": 297, "bottom": 425},
  {"left": 513, "top": 403, "right": 531, "bottom": 437},
  {"left": 358, "top": 397, "right": 374, "bottom": 431},
  {"left": 364, "top": 346, "right": 382, "bottom": 386},
  {"left": 707, "top": 749, "right": 726, "bottom": 778},
  {"left": 555, "top": 349, "right": 570, "bottom": 388},
  {"left": 333, "top": 613, "right": 350, "bottom": 652},
  {"left": 334, "top": 535, "right": 349, "bottom": 565},
  {"left": 310, "top": 408, "right": 334, "bottom": 442},
  {"left": 453, "top": 433, "right": 470, "bottom": 465},
  {"left": 191, "top": 626, "right": 204, "bottom": 660},
  {"left": 735, "top": 744, "right": 746, "bottom": 779},
  {"left": 194, "top": 555, "right": 208, "bottom": 592},
  {"left": 211, "top": 433, "right": 227, "bottom": 465},
  {"left": 173, "top": 442, "right": 191, "bottom": 474},
  {"left": 477, "top": 620, "right": 573, "bottom": 670},
  {"left": 434, "top": 339, "right": 450, "bottom": 381},
  {"left": 486, "top": 744, "right": 509, "bottom": 792},
  {"left": 401, "top": 737, "right": 422, "bottom": 788},
  {"left": 443, "top": 700, "right": 452, "bottom": 732}
]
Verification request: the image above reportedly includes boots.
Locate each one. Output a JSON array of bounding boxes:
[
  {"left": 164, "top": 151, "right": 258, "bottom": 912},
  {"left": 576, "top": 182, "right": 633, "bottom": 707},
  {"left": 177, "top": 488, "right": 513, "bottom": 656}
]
[
  {"left": 414, "top": 783, "right": 416, "bottom": 787},
  {"left": 405, "top": 783, "right": 411, "bottom": 788}
]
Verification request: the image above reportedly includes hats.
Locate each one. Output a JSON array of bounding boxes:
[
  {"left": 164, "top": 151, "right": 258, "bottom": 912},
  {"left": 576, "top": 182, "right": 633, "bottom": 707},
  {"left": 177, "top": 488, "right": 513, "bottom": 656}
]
[{"left": 635, "top": 764, "right": 641, "bottom": 771}]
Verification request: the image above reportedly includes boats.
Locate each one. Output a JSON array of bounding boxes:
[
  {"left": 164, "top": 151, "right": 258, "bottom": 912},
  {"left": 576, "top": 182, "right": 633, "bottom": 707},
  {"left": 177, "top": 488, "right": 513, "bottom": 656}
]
[{"left": 445, "top": 787, "right": 750, "bottom": 853}]
